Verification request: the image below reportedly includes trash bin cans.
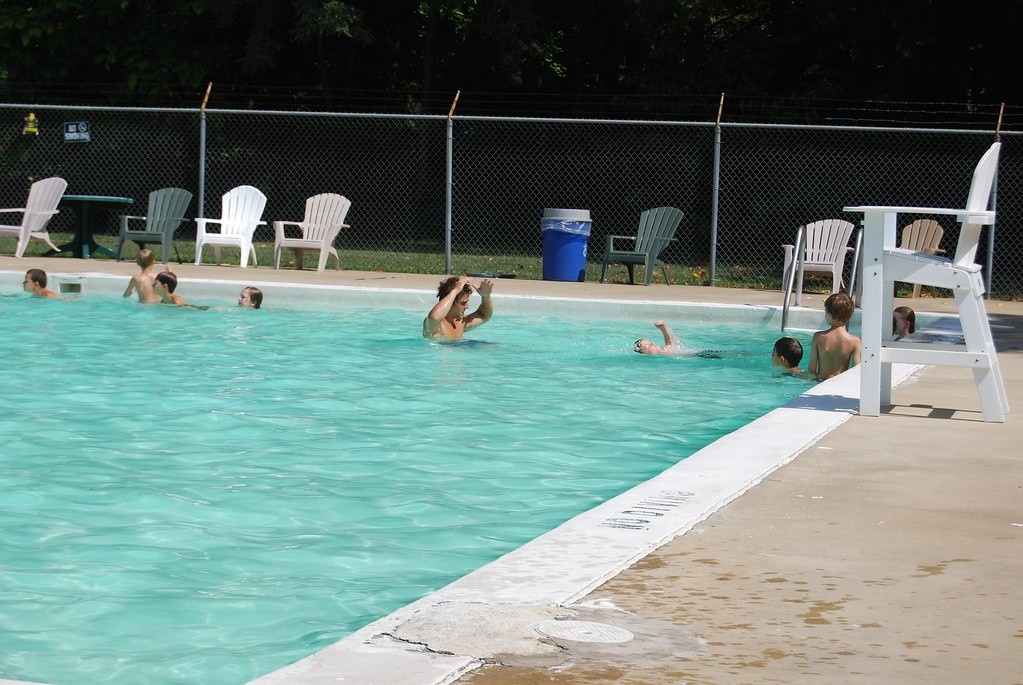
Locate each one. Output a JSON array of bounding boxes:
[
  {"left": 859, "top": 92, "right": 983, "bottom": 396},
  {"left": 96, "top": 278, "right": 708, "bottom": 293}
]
[{"left": 539, "top": 206, "right": 592, "bottom": 283}]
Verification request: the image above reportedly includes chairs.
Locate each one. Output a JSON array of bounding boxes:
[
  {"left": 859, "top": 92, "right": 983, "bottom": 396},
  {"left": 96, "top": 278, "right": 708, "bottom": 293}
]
[
  {"left": 600, "top": 207, "right": 684, "bottom": 287},
  {"left": 274, "top": 193, "right": 353, "bottom": 273},
  {"left": 781, "top": 218, "right": 856, "bottom": 307},
  {"left": 116, "top": 188, "right": 194, "bottom": 267},
  {"left": 1, "top": 177, "right": 69, "bottom": 259},
  {"left": 192, "top": 185, "right": 268, "bottom": 270},
  {"left": 841, "top": 142, "right": 1010, "bottom": 425},
  {"left": 896, "top": 218, "right": 946, "bottom": 299}
]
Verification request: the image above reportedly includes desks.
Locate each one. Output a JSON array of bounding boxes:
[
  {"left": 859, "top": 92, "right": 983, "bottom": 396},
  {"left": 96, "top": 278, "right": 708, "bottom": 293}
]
[{"left": 41, "top": 195, "right": 134, "bottom": 263}]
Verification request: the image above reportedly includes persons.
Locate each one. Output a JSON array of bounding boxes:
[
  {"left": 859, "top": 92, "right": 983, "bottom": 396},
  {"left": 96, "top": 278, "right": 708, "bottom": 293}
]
[
  {"left": 1, "top": 268, "right": 79, "bottom": 303},
  {"left": 238, "top": 286, "right": 263, "bottom": 310},
  {"left": 421, "top": 275, "right": 494, "bottom": 342},
  {"left": 893, "top": 316, "right": 896, "bottom": 335},
  {"left": 805, "top": 292, "right": 861, "bottom": 381},
  {"left": 635, "top": 320, "right": 770, "bottom": 362},
  {"left": 122, "top": 248, "right": 175, "bottom": 304},
  {"left": 893, "top": 305, "right": 916, "bottom": 341},
  {"left": 152, "top": 272, "right": 188, "bottom": 306},
  {"left": 772, "top": 337, "right": 804, "bottom": 377}
]
